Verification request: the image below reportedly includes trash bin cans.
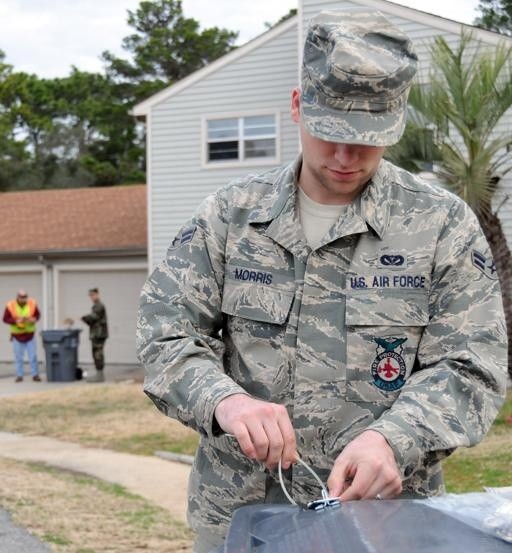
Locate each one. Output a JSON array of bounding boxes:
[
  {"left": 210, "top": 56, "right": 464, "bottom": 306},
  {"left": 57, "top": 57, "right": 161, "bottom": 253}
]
[{"left": 42, "top": 330, "right": 81, "bottom": 381}]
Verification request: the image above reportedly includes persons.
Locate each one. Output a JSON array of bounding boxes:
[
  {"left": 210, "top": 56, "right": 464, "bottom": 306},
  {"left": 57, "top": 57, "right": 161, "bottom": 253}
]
[
  {"left": 77, "top": 285, "right": 113, "bottom": 382},
  {"left": 131, "top": 4, "right": 510, "bottom": 552},
  {"left": 2, "top": 289, "right": 42, "bottom": 382}
]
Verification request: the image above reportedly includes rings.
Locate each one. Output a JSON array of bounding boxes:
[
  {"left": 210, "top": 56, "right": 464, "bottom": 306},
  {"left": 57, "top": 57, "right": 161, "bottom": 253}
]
[{"left": 374, "top": 494, "right": 382, "bottom": 501}]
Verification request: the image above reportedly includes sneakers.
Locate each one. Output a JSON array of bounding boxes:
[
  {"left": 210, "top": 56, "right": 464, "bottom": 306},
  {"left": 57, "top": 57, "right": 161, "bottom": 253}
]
[
  {"left": 14, "top": 376, "right": 24, "bottom": 383},
  {"left": 85, "top": 369, "right": 105, "bottom": 383},
  {"left": 33, "top": 375, "right": 42, "bottom": 382}
]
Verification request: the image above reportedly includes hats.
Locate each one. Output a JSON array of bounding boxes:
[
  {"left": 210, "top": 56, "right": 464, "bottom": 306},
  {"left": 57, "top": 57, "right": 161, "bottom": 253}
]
[
  {"left": 17, "top": 290, "right": 28, "bottom": 299},
  {"left": 88, "top": 286, "right": 99, "bottom": 296},
  {"left": 298, "top": 7, "right": 419, "bottom": 148}
]
